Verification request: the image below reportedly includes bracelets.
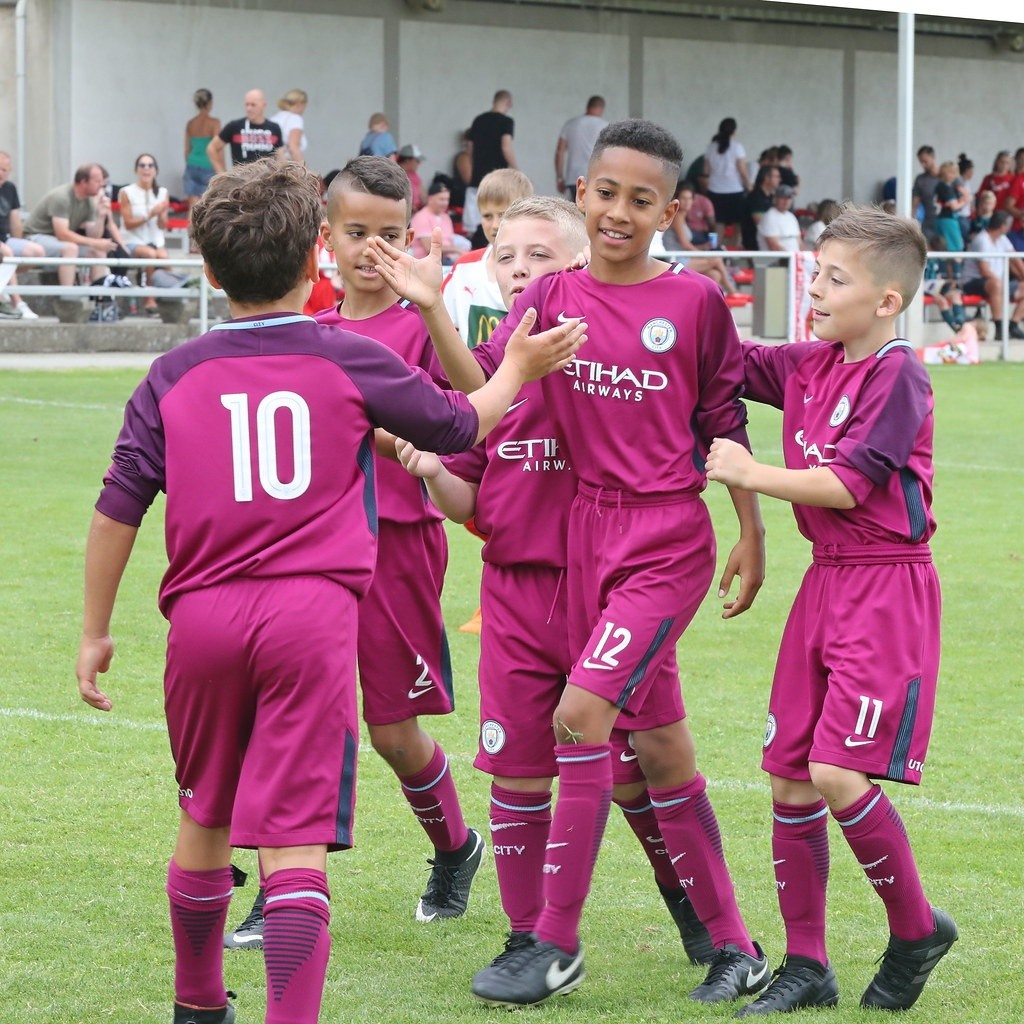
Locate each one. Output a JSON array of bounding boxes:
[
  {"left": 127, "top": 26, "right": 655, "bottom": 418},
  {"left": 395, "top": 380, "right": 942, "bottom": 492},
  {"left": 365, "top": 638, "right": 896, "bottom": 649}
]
[
  {"left": 143, "top": 213, "right": 149, "bottom": 221},
  {"left": 556, "top": 176, "right": 563, "bottom": 182}
]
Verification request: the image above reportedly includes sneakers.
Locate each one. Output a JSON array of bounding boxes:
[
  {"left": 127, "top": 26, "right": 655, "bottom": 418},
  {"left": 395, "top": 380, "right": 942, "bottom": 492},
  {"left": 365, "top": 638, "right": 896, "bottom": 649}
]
[
  {"left": 418, "top": 829, "right": 483, "bottom": 920},
  {"left": 859, "top": 908, "right": 959, "bottom": 1010},
  {"left": 220, "top": 905, "right": 266, "bottom": 948},
  {"left": 474, "top": 932, "right": 586, "bottom": 1011},
  {"left": 653, "top": 880, "right": 719, "bottom": 963},
  {"left": 692, "top": 943, "right": 767, "bottom": 1002},
  {"left": 475, "top": 932, "right": 539, "bottom": 987},
  {"left": 732, "top": 953, "right": 839, "bottom": 1017}
]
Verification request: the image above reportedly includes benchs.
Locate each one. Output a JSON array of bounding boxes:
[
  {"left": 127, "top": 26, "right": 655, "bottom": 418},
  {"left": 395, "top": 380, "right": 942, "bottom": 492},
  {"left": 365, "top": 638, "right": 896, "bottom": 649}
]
[{"left": 922, "top": 290, "right": 985, "bottom": 322}]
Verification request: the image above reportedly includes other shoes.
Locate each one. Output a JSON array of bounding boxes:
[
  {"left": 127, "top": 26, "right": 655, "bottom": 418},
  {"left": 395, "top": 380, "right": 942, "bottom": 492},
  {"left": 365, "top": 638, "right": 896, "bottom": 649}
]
[
  {"left": 14, "top": 302, "right": 39, "bottom": 320},
  {"left": 0, "top": 303, "right": 21, "bottom": 319},
  {"left": 1010, "top": 321, "right": 1024, "bottom": 339},
  {"left": 994, "top": 321, "right": 1003, "bottom": 340}
]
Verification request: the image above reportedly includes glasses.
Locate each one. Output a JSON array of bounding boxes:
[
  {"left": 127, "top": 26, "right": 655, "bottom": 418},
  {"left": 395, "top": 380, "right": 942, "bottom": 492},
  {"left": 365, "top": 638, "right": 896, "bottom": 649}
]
[{"left": 138, "top": 163, "right": 154, "bottom": 169}]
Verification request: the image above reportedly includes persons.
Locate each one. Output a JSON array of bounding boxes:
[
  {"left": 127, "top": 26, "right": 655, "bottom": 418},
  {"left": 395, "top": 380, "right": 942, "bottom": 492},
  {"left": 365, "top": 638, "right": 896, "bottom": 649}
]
[
  {"left": 910, "top": 146, "right": 1024, "bottom": 341},
  {"left": 117, "top": 153, "right": 171, "bottom": 317},
  {"left": 184, "top": 88, "right": 221, "bottom": 253},
  {"left": 77, "top": 159, "right": 588, "bottom": 1024},
  {"left": 207, "top": 90, "right": 284, "bottom": 176},
  {"left": 566, "top": 201, "right": 961, "bottom": 1018},
  {"left": 367, "top": 118, "right": 772, "bottom": 1005},
  {"left": 24, "top": 163, "right": 132, "bottom": 301},
  {"left": 452, "top": 89, "right": 518, "bottom": 232},
  {"left": 396, "top": 195, "right": 716, "bottom": 967},
  {"left": 553, "top": 96, "right": 609, "bottom": 202},
  {"left": 0, "top": 149, "right": 45, "bottom": 320},
  {"left": 661, "top": 117, "right": 837, "bottom": 295},
  {"left": 441, "top": 168, "right": 533, "bottom": 351},
  {"left": 223, "top": 155, "right": 486, "bottom": 951},
  {"left": 268, "top": 88, "right": 308, "bottom": 169},
  {"left": 360, "top": 110, "right": 466, "bottom": 266}
]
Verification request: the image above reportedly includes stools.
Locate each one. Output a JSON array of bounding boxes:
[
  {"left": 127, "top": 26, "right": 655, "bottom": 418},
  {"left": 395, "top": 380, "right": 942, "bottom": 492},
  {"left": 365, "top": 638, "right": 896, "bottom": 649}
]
[{"left": 108, "top": 201, "right": 191, "bottom": 254}]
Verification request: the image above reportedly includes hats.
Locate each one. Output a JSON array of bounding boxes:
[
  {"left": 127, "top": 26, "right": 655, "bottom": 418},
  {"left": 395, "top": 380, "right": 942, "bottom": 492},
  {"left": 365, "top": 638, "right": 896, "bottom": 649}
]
[
  {"left": 428, "top": 183, "right": 447, "bottom": 195},
  {"left": 775, "top": 185, "right": 797, "bottom": 197},
  {"left": 399, "top": 145, "right": 426, "bottom": 163}
]
